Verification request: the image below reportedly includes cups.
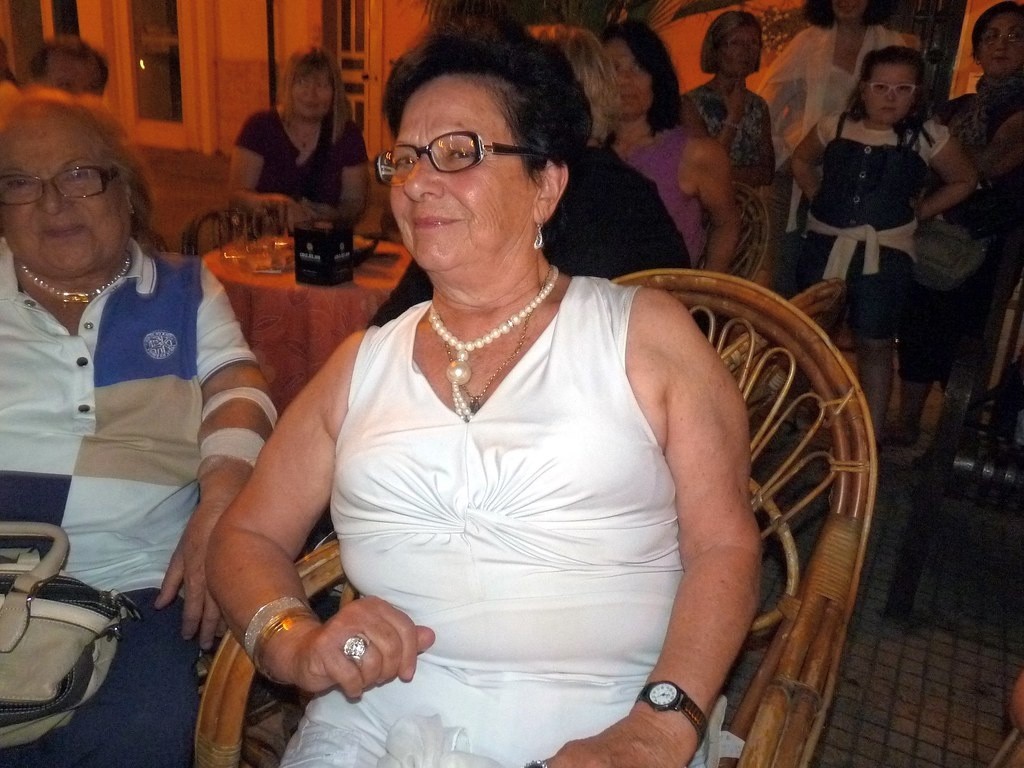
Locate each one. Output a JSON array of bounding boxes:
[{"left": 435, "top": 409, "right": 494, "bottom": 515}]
[{"left": 219, "top": 202, "right": 302, "bottom": 275}]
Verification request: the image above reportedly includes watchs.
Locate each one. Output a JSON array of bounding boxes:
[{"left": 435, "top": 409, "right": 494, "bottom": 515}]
[{"left": 636, "top": 680, "right": 707, "bottom": 748}]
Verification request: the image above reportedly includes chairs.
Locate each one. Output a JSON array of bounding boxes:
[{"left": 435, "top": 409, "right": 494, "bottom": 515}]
[
  {"left": 194, "top": 262, "right": 880, "bottom": 767},
  {"left": 704, "top": 179, "right": 778, "bottom": 287},
  {"left": 717, "top": 276, "right": 854, "bottom": 636},
  {"left": 886, "top": 241, "right": 1024, "bottom": 621}
]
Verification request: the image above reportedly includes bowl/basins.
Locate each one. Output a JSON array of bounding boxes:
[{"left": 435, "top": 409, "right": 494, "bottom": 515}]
[{"left": 353, "top": 234, "right": 379, "bottom": 270}]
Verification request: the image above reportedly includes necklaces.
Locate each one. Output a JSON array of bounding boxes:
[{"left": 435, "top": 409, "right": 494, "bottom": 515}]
[
  {"left": 20, "top": 258, "right": 131, "bottom": 303},
  {"left": 428, "top": 267, "right": 559, "bottom": 425},
  {"left": 445, "top": 265, "right": 558, "bottom": 423}
]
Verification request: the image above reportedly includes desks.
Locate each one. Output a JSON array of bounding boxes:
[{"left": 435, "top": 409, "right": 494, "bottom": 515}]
[{"left": 191, "top": 236, "right": 419, "bottom": 402}]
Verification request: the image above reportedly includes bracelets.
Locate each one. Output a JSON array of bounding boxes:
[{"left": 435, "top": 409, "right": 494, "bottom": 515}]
[
  {"left": 245, "top": 595, "right": 313, "bottom": 685},
  {"left": 724, "top": 120, "right": 741, "bottom": 130}
]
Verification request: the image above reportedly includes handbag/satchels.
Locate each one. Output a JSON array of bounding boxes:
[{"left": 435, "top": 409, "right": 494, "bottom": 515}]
[
  {"left": 0, "top": 519, "right": 139, "bottom": 744},
  {"left": 914, "top": 216, "right": 990, "bottom": 293}
]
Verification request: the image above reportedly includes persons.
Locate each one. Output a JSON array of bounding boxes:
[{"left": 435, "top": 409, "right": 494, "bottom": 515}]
[
  {"left": 203, "top": 14, "right": 764, "bottom": 768},
  {"left": 227, "top": 46, "right": 370, "bottom": 238},
  {"left": 0, "top": 97, "right": 277, "bottom": 768},
  {"left": 31, "top": 37, "right": 110, "bottom": 96},
  {"left": 368, "top": 0, "right": 1024, "bottom": 449}
]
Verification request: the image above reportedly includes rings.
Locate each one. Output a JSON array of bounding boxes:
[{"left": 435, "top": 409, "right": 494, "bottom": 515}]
[
  {"left": 524, "top": 760, "right": 548, "bottom": 768},
  {"left": 343, "top": 633, "right": 371, "bottom": 661}
]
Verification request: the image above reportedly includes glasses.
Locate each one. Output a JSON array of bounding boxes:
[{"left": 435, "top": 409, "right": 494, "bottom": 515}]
[
  {"left": 864, "top": 81, "right": 918, "bottom": 100},
  {"left": 0, "top": 166, "right": 118, "bottom": 206},
  {"left": 375, "top": 129, "right": 563, "bottom": 188},
  {"left": 981, "top": 29, "right": 1024, "bottom": 49}
]
